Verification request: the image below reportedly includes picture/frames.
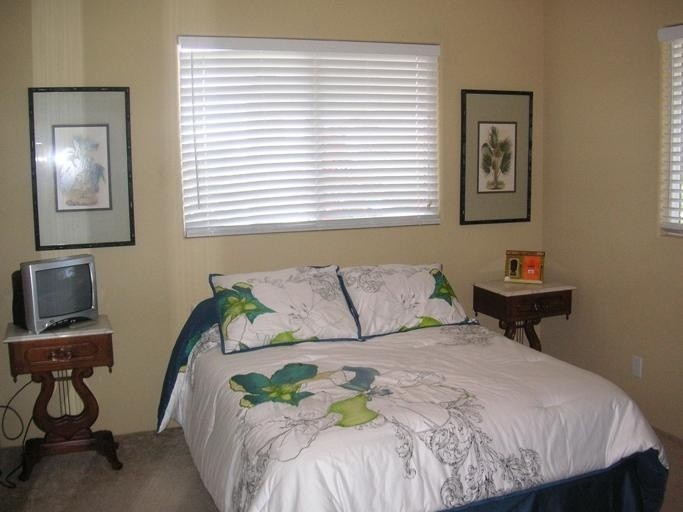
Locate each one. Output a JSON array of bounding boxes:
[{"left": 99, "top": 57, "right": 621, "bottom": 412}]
[
  {"left": 460, "top": 89, "right": 533, "bottom": 225},
  {"left": 27, "top": 87, "right": 135, "bottom": 251}
]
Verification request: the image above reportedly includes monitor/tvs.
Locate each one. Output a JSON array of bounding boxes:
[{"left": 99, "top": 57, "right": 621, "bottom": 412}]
[{"left": 11, "top": 253, "right": 99, "bottom": 334}]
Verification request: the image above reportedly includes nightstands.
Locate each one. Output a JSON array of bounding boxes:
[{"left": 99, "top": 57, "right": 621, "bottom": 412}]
[
  {"left": 472, "top": 278, "right": 576, "bottom": 352},
  {"left": 2, "top": 315, "right": 124, "bottom": 482}
]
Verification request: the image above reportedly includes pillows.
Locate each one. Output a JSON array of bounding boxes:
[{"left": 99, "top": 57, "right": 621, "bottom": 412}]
[{"left": 208, "top": 263, "right": 480, "bottom": 354}]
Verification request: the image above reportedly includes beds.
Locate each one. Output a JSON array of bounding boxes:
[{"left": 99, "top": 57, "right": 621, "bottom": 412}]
[{"left": 172, "top": 296, "right": 652, "bottom": 512}]
